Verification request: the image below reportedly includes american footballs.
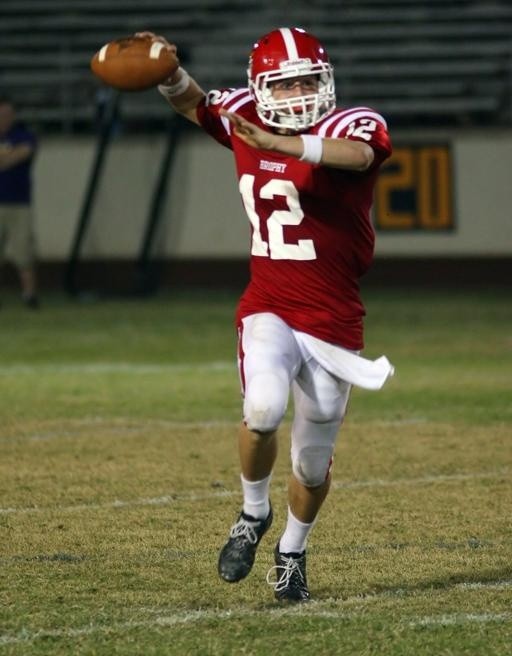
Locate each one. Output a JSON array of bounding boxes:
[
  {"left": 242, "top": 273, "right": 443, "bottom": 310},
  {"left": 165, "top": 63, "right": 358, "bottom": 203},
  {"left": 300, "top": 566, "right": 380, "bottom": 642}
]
[{"left": 91, "top": 36, "right": 179, "bottom": 92}]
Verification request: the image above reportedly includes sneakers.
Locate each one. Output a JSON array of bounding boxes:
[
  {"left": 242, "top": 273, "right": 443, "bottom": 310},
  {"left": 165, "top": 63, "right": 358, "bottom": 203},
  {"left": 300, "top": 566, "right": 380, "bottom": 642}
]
[
  {"left": 273, "top": 540, "right": 312, "bottom": 603},
  {"left": 216, "top": 499, "right": 276, "bottom": 583}
]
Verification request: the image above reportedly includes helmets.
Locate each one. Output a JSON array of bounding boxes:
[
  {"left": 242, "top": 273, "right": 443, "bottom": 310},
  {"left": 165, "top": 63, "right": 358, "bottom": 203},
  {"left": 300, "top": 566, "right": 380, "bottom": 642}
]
[{"left": 245, "top": 25, "right": 337, "bottom": 133}]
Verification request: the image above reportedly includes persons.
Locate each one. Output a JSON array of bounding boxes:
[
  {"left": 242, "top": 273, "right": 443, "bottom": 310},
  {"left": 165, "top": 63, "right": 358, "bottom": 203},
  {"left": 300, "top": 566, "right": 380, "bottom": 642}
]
[
  {"left": 0, "top": 96, "right": 42, "bottom": 310},
  {"left": 129, "top": 25, "right": 394, "bottom": 604}
]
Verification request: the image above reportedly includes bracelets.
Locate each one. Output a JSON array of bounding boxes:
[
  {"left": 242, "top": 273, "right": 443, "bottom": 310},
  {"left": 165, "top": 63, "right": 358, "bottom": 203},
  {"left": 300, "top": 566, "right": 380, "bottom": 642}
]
[
  {"left": 156, "top": 68, "right": 191, "bottom": 98},
  {"left": 296, "top": 133, "right": 323, "bottom": 165}
]
